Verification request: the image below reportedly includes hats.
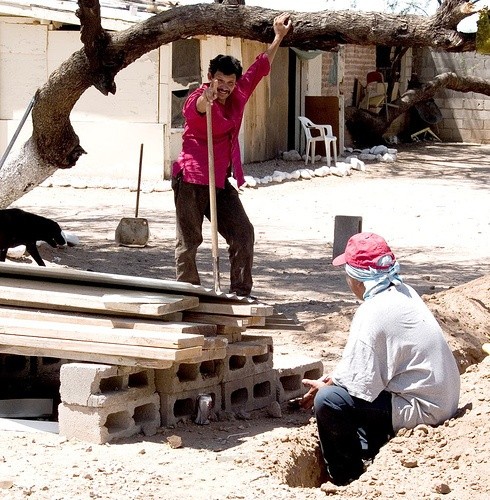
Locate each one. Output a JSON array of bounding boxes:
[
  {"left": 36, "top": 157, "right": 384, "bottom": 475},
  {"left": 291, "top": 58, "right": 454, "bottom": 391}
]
[{"left": 332, "top": 231, "right": 396, "bottom": 270}]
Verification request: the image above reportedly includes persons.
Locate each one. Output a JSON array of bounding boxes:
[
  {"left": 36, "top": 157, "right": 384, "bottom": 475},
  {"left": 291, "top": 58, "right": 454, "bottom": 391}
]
[
  {"left": 170, "top": 12, "right": 293, "bottom": 300},
  {"left": 298, "top": 232, "right": 461, "bottom": 492}
]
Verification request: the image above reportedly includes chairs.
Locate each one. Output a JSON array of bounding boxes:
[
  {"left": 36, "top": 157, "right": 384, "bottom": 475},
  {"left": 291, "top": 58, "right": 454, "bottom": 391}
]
[{"left": 298, "top": 116, "right": 338, "bottom": 167}]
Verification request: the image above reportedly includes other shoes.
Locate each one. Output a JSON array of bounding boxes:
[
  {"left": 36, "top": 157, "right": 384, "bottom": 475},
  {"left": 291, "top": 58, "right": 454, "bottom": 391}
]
[{"left": 246, "top": 296, "right": 258, "bottom": 301}]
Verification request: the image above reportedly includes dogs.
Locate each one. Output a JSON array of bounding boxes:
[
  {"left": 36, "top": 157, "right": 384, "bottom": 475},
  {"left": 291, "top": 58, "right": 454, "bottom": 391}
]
[{"left": 0, "top": 208, "right": 66, "bottom": 266}]
